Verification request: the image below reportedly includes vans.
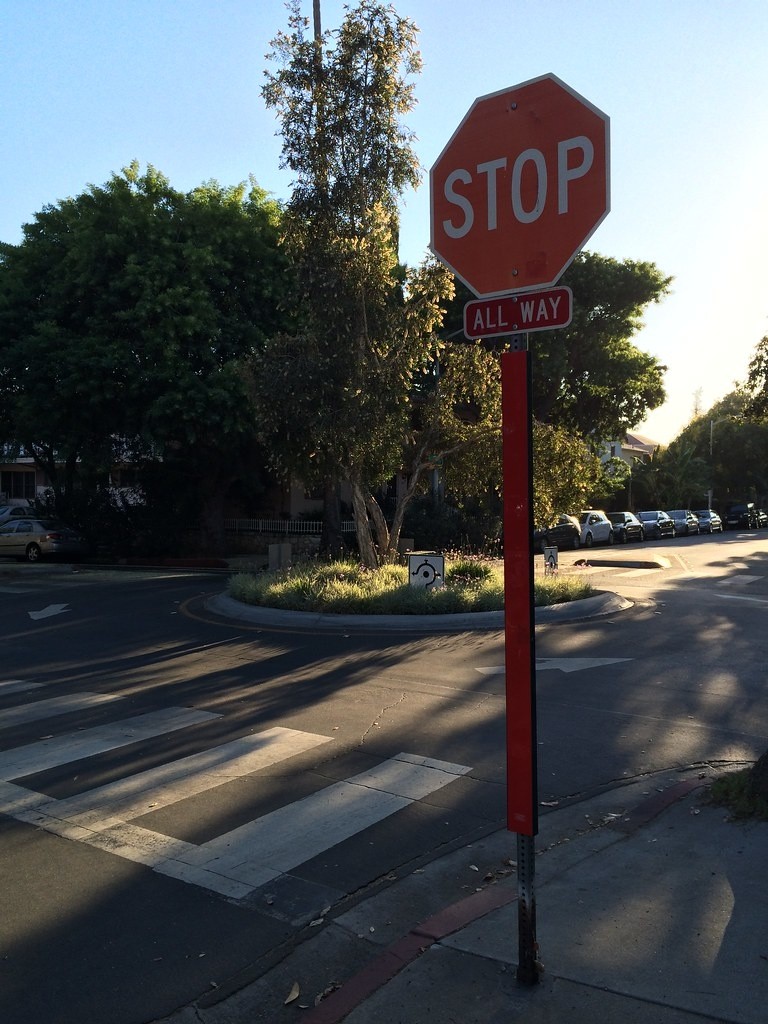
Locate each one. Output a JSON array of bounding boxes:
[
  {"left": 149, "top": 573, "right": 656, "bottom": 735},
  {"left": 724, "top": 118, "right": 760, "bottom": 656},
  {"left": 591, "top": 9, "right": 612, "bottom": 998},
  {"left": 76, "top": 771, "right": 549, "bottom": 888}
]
[{"left": 722, "top": 501, "right": 756, "bottom": 531}]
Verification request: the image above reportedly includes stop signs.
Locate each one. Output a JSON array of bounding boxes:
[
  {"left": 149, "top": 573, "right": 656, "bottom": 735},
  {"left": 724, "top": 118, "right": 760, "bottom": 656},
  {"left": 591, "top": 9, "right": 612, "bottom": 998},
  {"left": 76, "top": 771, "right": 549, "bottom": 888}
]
[{"left": 431, "top": 68, "right": 612, "bottom": 995}]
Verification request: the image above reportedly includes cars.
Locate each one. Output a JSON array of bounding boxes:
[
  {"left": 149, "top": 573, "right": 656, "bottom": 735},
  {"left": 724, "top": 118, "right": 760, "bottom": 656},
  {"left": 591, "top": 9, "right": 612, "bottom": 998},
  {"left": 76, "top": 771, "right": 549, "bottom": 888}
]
[
  {"left": 576, "top": 509, "right": 613, "bottom": 548},
  {"left": 635, "top": 511, "right": 676, "bottom": 540},
  {"left": 755, "top": 509, "right": 768, "bottom": 530},
  {"left": 692, "top": 509, "right": 722, "bottom": 533},
  {"left": 605, "top": 512, "right": 644, "bottom": 544},
  {"left": 0, "top": 519, "right": 81, "bottom": 563},
  {"left": 666, "top": 510, "right": 700, "bottom": 536},
  {"left": 534, "top": 512, "right": 581, "bottom": 554}
]
